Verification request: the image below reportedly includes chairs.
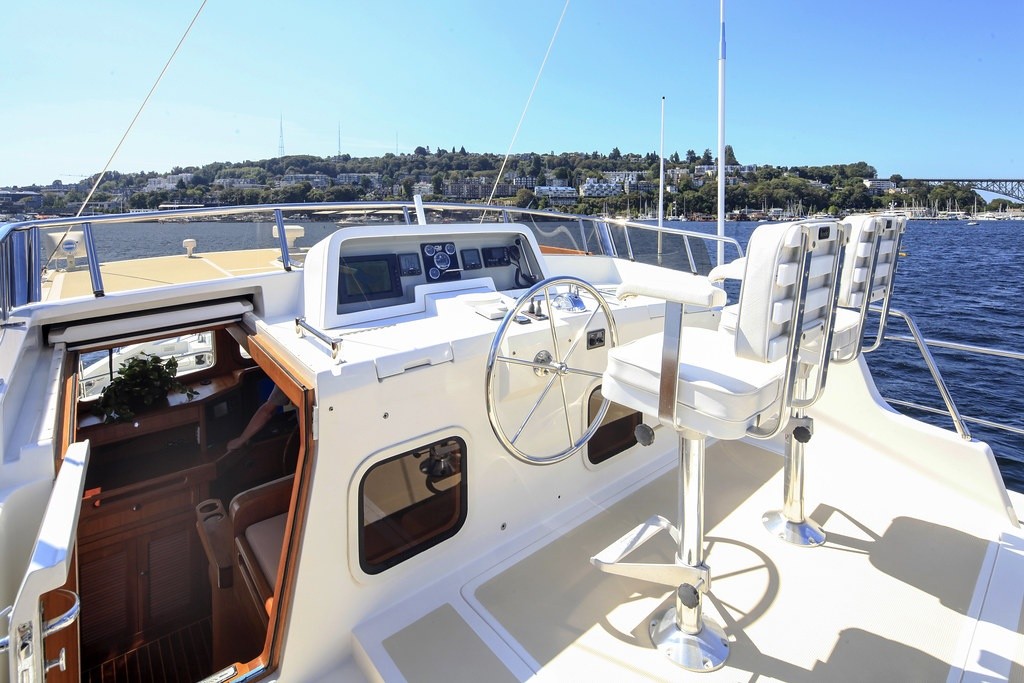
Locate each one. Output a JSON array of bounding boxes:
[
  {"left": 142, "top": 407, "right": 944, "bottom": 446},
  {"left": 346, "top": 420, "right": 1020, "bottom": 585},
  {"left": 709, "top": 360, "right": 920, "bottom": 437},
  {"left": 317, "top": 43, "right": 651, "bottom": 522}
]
[
  {"left": 706, "top": 214, "right": 907, "bottom": 548},
  {"left": 588, "top": 218, "right": 852, "bottom": 673}
]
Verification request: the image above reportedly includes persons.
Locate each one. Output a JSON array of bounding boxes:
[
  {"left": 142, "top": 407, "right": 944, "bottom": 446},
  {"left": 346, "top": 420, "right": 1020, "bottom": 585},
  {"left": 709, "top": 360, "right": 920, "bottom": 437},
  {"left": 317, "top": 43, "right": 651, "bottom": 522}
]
[{"left": 226, "top": 383, "right": 300, "bottom": 450}]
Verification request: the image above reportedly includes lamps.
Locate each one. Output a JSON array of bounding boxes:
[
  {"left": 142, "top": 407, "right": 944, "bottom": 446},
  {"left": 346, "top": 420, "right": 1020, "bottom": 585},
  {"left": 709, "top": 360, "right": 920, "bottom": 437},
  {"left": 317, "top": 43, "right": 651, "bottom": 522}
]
[{"left": 428, "top": 441, "right": 460, "bottom": 477}]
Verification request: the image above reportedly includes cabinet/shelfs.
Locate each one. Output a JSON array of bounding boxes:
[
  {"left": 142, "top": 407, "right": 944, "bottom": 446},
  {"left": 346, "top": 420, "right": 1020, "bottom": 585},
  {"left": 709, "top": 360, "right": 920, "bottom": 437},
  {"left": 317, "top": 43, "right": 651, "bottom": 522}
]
[{"left": 76, "top": 480, "right": 211, "bottom": 670}]
